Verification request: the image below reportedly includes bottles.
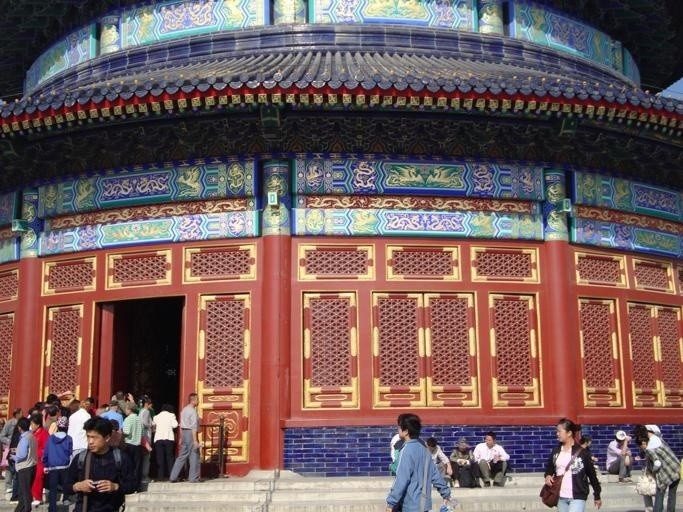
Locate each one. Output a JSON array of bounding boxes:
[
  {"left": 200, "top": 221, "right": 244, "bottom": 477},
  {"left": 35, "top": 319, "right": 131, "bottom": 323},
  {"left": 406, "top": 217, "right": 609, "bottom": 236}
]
[{"left": 439, "top": 498, "right": 459, "bottom": 512}]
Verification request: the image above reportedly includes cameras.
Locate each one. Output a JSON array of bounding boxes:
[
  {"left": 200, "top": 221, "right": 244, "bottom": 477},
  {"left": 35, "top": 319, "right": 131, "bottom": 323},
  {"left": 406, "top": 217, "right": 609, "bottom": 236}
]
[{"left": 91, "top": 482, "right": 100, "bottom": 487}]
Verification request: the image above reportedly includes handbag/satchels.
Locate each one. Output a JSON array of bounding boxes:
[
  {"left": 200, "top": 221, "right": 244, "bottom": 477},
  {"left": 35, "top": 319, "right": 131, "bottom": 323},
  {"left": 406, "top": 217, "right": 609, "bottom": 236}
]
[
  {"left": 0, "top": 441, "right": 11, "bottom": 466},
  {"left": 635, "top": 473, "right": 658, "bottom": 496},
  {"left": 539, "top": 475, "right": 564, "bottom": 508},
  {"left": 587, "top": 464, "right": 604, "bottom": 485}
]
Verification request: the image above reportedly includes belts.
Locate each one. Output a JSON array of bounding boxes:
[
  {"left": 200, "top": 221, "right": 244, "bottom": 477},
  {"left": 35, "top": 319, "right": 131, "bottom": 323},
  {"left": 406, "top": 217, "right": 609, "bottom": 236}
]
[{"left": 180, "top": 428, "right": 192, "bottom": 430}]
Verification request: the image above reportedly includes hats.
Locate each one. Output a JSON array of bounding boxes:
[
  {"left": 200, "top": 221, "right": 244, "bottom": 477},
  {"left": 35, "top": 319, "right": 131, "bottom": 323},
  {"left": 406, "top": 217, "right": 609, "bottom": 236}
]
[
  {"left": 615, "top": 430, "right": 626, "bottom": 440},
  {"left": 455, "top": 438, "right": 470, "bottom": 448},
  {"left": 56, "top": 416, "right": 69, "bottom": 428}
]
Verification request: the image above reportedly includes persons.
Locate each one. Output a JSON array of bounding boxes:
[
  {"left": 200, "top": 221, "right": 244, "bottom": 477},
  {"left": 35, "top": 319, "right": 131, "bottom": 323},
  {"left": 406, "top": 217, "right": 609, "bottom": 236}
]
[
  {"left": 385, "top": 413, "right": 451, "bottom": 512},
  {"left": 449, "top": 438, "right": 481, "bottom": 488},
  {"left": 389, "top": 434, "right": 405, "bottom": 474},
  {"left": 544, "top": 419, "right": 602, "bottom": 512},
  {"left": 424, "top": 437, "right": 453, "bottom": 486},
  {"left": 473, "top": 432, "right": 511, "bottom": 487},
  {"left": 580, "top": 424, "right": 681, "bottom": 512},
  {"left": 0, "top": 391, "right": 204, "bottom": 512}
]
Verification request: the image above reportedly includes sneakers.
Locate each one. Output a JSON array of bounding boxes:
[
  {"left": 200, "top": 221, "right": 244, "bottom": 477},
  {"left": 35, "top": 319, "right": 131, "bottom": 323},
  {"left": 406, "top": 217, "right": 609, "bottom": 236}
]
[
  {"left": 484, "top": 480, "right": 490, "bottom": 487},
  {"left": 189, "top": 478, "right": 205, "bottom": 482},
  {"left": 492, "top": 481, "right": 504, "bottom": 487},
  {"left": 5, "top": 487, "right": 73, "bottom": 507},
  {"left": 618, "top": 476, "right": 633, "bottom": 483},
  {"left": 170, "top": 478, "right": 185, "bottom": 483}
]
